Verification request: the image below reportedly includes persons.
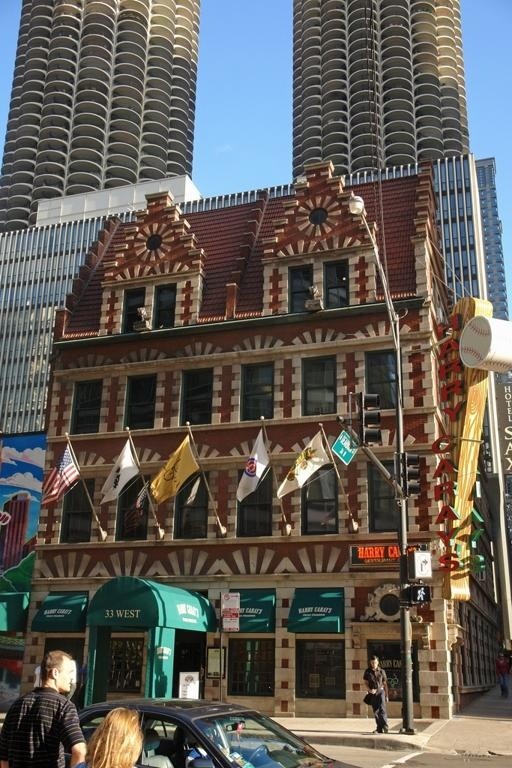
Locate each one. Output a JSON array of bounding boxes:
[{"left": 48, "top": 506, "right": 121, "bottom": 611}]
[
  {"left": 495, "top": 648, "right": 510, "bottom": 698},
  {"left": 362, "top": 655, "right": 388, "bottom": 733},
  {"left": 184, "top": 723, "right": 245, "bottom": 768},
  {"left": 0, "top": 650, "right": 87, "bottom": 768},
  {"left": 71, "top": 706, "right": 146, "bottom": 768}
]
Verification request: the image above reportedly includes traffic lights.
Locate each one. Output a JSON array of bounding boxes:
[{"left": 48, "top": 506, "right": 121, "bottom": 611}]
[
  {"left": 407, "top": 452, "right": 421, "bottom": 495},
  {"left": 413, "top": 587, "right": 430, "bottom": 601},
  {"left": 365, "top": 393, "right": 383, "bottom": 442},
  {"left": 380, "top": 595, "right": 401, "bottom": 616}
]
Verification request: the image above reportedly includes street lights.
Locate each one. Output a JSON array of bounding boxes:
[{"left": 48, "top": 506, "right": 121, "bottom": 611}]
[{"left": 348, "top": 190, "right": 418, "bottom": 735}]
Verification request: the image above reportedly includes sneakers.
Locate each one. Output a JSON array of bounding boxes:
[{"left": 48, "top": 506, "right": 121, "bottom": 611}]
[{"left": 373, "top": 725, "right": 389, "bottom": 734}]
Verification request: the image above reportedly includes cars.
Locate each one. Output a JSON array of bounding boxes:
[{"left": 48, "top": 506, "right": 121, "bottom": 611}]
[{"left": 56, "top": 698, "right": 362, "bottom": 768}]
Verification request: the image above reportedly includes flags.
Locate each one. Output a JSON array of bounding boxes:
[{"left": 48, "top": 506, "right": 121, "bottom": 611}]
[
  {"left": 40, "top": 442, "right": 81, "bottom": 506},
  {"left": 150, "top": 434, "right": 201, "bottom": 503},
  {"left": 99, "top": 437, "right": 140, "bottom": 504},
  {"left": 235, "top": 428, "right": 270, "bottom": 502},
  {"left": 276, "top": 430, "right": 331, "bottom": 499}
]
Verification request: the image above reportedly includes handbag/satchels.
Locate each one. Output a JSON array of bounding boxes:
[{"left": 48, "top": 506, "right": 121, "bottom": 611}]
[{"left": 363, "top": 692, "right": 379, "bottom": 706}]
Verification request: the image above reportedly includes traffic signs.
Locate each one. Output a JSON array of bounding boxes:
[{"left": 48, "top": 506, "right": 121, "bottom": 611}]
[{"left": 331, "top": 431, "right": 357, "bottom": 466}]
